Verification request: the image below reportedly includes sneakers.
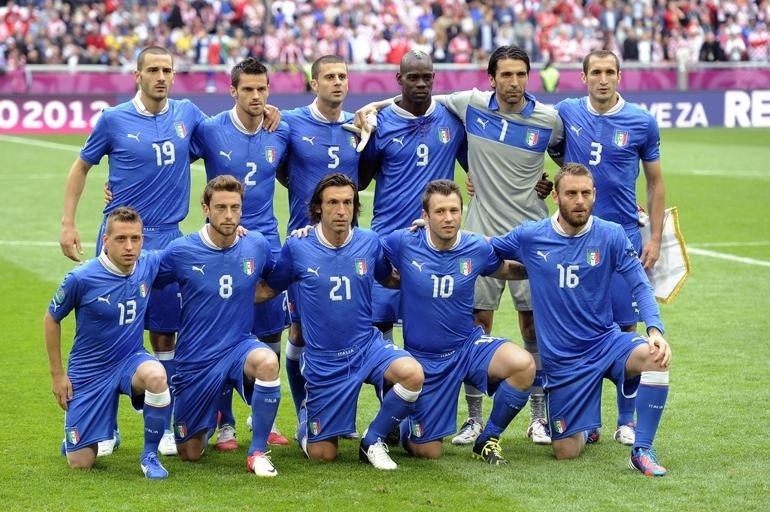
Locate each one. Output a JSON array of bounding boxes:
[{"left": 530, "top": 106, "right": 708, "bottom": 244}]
[
  {"left": 246, "top": 414, "right": 289, "bottom": 477},
  {"left": 214, "top": 423, "right": 238, "bottom": 451},
  {"left": 360, "top": 428, "right": 397, "bottom": 470},
  {"left": 96, "top": 428, "right": 121, "bottom": 458},
  {"left": 451, "top": 417, "right": 509, "bottom": 466},
  {"left": 140, "top": 430, "right": 178, "bottom": 479},
  {"left": 587, "top": 424, "right": 667, "bottom": 477},
  {"left": 526, "top": 418, "right": 552, "bottom": 445}
]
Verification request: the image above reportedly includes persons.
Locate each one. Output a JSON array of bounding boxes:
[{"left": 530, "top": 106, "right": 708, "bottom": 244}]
[
  {"left": 291, "top": 178, "right": 536, "bottom": 468},
  {"left": 467, "top": 45, "right": 664, "bottom": 443},
  {"left": 105, "top": 57, "right": 290, "bottom": 453},
  {"left": 412, "top": 161, "right": 671, "bottom": 476},
  {"left": 360, "top": 51, "right": 553, "bottom": 444},
  {"left": 43, "top": 205, "right": 249, "bottom": 479},
  {"left": 151, "top": 174, "right": 311, "bottom": 479},
  {"left": 353, "top": 51, "right": 644, "bottom": 449},
  {"left": 255, "top": 172, "right": 424, "bottom": 470},
  {"left": 279, "top": 54, "right": 369, "bottom": 442},
  {"left": 0, "top": 1, "right": 769, "bottom": 72},
  {"left": 59, "top": 44, "right": 281, "bottom": 457}
]
